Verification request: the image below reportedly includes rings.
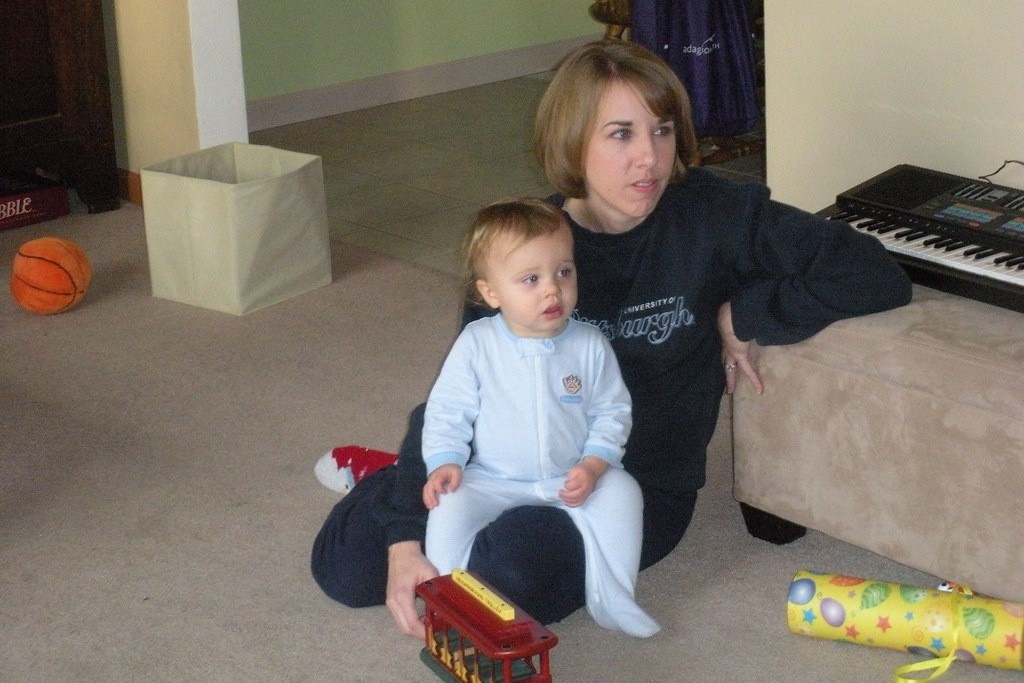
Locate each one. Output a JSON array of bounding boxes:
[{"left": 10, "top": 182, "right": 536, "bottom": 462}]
[{"left": 724, "top": 362, "right": 738, "bottom": 369}]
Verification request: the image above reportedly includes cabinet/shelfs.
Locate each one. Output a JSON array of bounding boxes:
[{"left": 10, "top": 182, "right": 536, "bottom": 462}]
[{"left": 0, "top": 0, "right": 122, "bottom": 215}]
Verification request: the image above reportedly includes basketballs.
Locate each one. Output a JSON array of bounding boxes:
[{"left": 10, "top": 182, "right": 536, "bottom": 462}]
[{"left": 10, "top": 235, "right": 94, "bottom": 318}]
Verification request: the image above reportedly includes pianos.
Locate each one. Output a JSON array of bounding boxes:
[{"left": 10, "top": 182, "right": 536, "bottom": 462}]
[{"left": 810, "top": 160, "right": 1024, "bottom": 316}]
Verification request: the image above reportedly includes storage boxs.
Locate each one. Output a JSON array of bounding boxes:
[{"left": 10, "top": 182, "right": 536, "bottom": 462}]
[
  {"left": 140, "top": 142, "right": 332, "bottom": 316},
  {"left": 0, "top": 172, "right": 70, "bottom": 230}
]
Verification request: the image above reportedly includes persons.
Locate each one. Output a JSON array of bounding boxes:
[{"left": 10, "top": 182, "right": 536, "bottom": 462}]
[
  {"left": 421, "top": 196, "right": 661, "bottom": 639},
  {"left": 310, "top": 39, "right": 913, "bottom": 642}
]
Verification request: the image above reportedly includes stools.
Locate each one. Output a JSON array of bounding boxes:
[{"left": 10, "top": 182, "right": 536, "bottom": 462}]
[
  {"left": 728, "top": 281, "right": 1024, "bottom": 603},
  {"left": 589, "top": 0, "right": 763, "bottom": 166}
]
